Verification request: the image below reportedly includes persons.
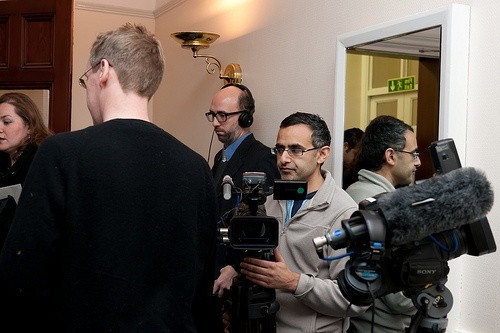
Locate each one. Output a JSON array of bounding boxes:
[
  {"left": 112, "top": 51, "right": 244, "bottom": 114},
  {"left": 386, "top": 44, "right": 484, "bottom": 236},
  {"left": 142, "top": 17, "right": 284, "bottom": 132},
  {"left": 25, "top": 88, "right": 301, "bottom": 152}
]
[
  {"left": 206, "top": 82, "right": 275, "bottom": 295},
  {"left": 0, "top": 22, "right": 219, "bottom": 333},
  {"left": 240, "top": 111, "right": 374, "bottom": 332},
  {"left": 343, "top": 114, "right": 420, "bottom": 332}
]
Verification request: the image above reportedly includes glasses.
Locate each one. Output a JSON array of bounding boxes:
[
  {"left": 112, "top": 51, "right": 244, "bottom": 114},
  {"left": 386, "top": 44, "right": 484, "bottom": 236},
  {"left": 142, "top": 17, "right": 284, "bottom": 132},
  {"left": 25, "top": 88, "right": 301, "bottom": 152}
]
[
  {"left": 204, "top": 110, "right": 246, "bottom": 122},
  {"left": 78, "top": 61, "right": 113, "bottom": 89},
  {"left": 270, "top": 144, "right": 326, "bottom": 157},
  {"left": 394, "top": 148, "right": 420, "bottom": 159}
]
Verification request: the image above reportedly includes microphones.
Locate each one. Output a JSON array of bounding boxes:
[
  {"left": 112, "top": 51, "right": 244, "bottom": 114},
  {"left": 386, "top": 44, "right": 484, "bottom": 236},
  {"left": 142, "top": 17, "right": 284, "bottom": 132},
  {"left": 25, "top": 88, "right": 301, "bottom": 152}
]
[
  {"left": 312, "top": 167, "right": 494, "bottom": 247},
  {"left": 223, "top": 175, "right": 231, "bottom": 200}
]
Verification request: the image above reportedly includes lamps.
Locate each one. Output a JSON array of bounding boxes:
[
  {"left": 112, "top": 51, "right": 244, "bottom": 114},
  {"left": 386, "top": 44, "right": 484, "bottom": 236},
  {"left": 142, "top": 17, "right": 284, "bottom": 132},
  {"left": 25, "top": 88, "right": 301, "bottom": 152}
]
[{"left": 170, "top": 30, "right": 242, "bottom": 85}]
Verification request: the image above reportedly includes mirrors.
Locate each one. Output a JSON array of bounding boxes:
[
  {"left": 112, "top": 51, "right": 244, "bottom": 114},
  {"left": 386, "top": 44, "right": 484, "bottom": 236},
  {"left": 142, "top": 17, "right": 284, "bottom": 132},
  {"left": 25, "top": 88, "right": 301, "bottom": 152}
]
[{"left": 326, "top": 3, "right": 470, "bottom": 190}]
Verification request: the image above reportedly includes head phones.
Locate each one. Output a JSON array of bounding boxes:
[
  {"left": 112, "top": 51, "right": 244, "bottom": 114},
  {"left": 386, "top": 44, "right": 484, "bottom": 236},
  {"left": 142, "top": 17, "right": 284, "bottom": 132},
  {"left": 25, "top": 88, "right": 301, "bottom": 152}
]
[{"left": 220, "top": 83, "right": 254, "bottom": 128}]
